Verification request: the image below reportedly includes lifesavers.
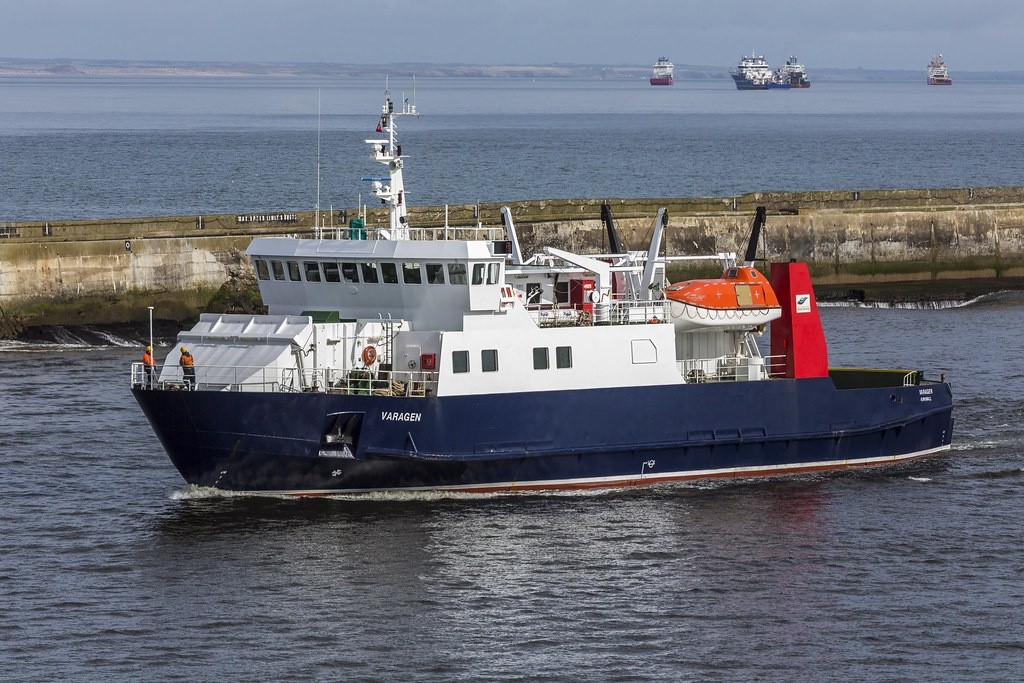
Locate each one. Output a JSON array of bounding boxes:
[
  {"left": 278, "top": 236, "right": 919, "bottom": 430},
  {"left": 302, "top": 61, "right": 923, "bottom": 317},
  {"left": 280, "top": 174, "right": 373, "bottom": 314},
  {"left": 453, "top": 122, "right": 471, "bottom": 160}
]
[{"left": 362, "top": 346, "right": 377, "bottom": 365}]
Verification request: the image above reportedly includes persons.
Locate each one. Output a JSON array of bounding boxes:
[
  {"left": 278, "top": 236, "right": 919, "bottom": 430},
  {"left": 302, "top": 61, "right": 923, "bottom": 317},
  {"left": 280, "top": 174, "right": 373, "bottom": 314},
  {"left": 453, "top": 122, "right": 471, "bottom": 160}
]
[
  {"left": 179, "top": 346, "right": 196, "bottom": 391},
  {"left": 143, "top": 346, "right": 157, "bottom": 383}
]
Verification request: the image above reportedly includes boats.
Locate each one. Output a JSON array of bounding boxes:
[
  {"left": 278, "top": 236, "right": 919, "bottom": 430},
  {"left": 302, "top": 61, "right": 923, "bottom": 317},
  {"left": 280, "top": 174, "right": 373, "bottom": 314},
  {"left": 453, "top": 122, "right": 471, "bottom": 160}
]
[
  {"left": 649, "top": 57, "right": 674, "bottom": 85},
  {"left": 728, "top": 50, "right": 790, "bottom": 89},
  {"left": 926, "top": 53, "right": 953, "bottom": 85},
  {"left": 781, "top": 56, "right": 810, "bottom": 87},
  {"left": 125, "top": 75, "right": 957, "bottom": 500}
]
